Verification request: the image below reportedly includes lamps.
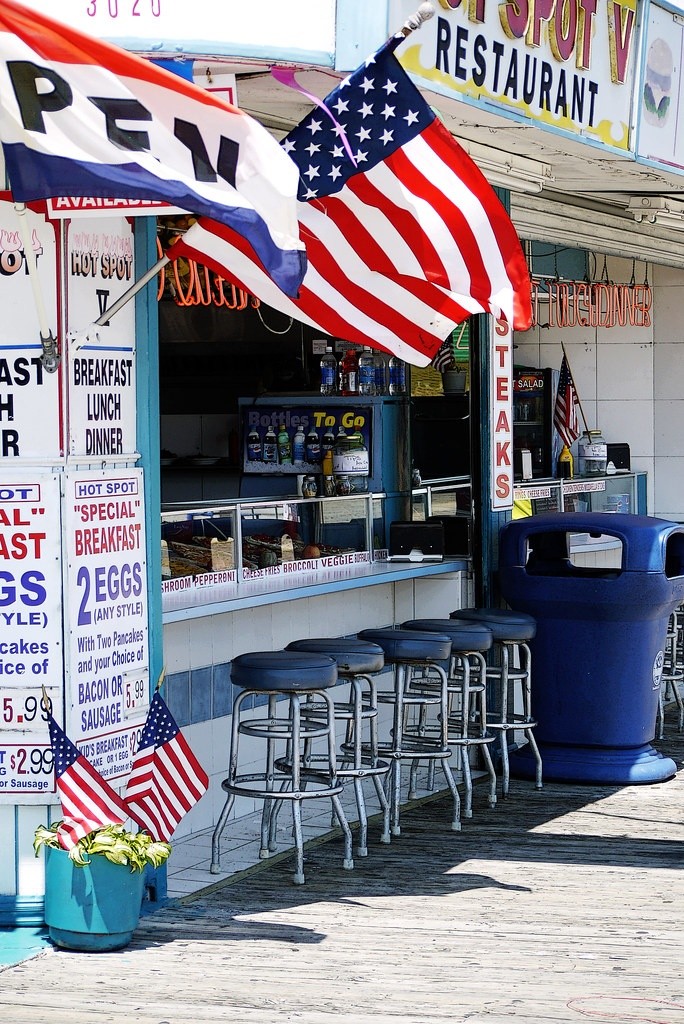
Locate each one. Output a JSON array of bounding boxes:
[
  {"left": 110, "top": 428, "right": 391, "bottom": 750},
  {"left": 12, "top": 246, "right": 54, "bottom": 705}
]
[
  {"left": 449, "top": 134, "right": 554, "bottom": 196},
  {"left": 624, "top": 196, "right": 684, "bottom": 231}
]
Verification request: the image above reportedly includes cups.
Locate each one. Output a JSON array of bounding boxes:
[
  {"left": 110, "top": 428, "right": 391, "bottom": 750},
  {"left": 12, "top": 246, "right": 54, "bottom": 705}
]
[
  {"left": 513, "top": 405, "right": 517, "bottom": 422},
  {"left": 518, "top": 403, "right": 535, "bottom": 420},
  {"left": 535, "top": 397, "right": 543, "bottom": 422}
]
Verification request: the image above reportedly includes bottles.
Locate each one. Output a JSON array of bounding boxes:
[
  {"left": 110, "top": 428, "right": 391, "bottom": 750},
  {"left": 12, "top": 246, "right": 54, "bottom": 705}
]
[
  {"left": 411, "top": 469, "right": 421, "bottom": 488},
  {"left": 276, "top": 424, "right": 292, "bottom": 465},
  {"left": 261, "top": 425, "right": 277, "bottom": 464},
  {"left": 321, "top": 426, "right": 335, "bottom": 450},
  {"left": 342, "top": 349, "right": 359, "bottom": 396},
  {"left": 559, "top": 444, "right": 574, "bottom": 478},
  {"left": 319, "top": 347, "right": 337, "bottom": 397},
  {"left": 338, "top": 350, "right": 346, "bottom": 394},
  {"left": 388, "top": 355, "right": 407, "bottom": 396},
  {"left": 578, "top": 430, "right": 608, "bottom": 478},
  {"left": 372, "top": 349, "right": 387, "bottom": 394},
  {"left": 306, "top": 425, "right": 321, "bottom": 466},
  {"left": 336, "top": 426, "right": 348, "bottom": 436},
  {"left": 324, "top": 475, "right": 351, "bottom": 497},
  {"left": 332, "top": 435, "right": 370, "bottom": 476},
  {"left": 322, "top": 450, "right": 333, "bottom": 476},
  {"left": 302, "top": 476, "right": 317, "bottom": 497},
  {"left": 292, "top": 425, "right": 306, "bottom": 465},
  {"left": 246, "top": 424, "right": 261, "bottom": 461},
  {"left": 352, "top": 425, "right": 365, "bottom": 445},
  {"left": 357, "top": 345, "right": 377, "bottom": 397}
]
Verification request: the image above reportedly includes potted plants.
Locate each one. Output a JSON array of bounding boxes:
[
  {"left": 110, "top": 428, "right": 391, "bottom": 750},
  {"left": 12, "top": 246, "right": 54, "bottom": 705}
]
[
  {"left": 442, "top": 365, "right": 467, "bottom": 393},
  {"left": 33, "top": 820, "right": 174, "bottom": 953}
]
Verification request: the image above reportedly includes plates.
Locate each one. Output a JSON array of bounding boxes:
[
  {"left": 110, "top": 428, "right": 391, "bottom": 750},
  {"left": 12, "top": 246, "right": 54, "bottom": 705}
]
[
  {"left": 190, "top": 457, "right": 221, "bottom": 465},
  {"left": 160, "top": 458, "right": 177, "bottom": 466}
]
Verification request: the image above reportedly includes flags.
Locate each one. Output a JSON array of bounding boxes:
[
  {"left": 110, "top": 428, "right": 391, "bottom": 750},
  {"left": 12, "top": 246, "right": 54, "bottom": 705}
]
[
  {"left": 165, "top": 44, "right": 532, "bottom": 369},
  {"left": 124, "top": 668, "right": 209, "bottom": 844},
  {"left": 0, "top": 0, "right": 308, "bottom": 299},
  {"left": 553, "top": 341, "right": 581, "bottom": 449},
  {"left": 42, "top": 684, "right": 155, "bottom": 851}
]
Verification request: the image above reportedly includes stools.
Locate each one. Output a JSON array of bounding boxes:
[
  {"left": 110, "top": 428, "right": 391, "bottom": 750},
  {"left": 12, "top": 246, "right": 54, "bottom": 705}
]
[
  {"left": 210, "top": 608, "right": 537, "bottom": 884},
  {"left": 658, "top": 601, "right": 684, "bottom": 739}
]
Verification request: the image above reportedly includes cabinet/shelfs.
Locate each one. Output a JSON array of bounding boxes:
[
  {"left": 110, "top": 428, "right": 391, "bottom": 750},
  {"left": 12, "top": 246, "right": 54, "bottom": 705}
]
[{"left": 237, "top": 395, "right": 399, "bottom": 555}]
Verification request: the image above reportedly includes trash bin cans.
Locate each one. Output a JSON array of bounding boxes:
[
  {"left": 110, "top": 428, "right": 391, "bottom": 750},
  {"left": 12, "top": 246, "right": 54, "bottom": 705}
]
[{"left": 494, "top": 512, "right": 684, "bottom": 784}]
